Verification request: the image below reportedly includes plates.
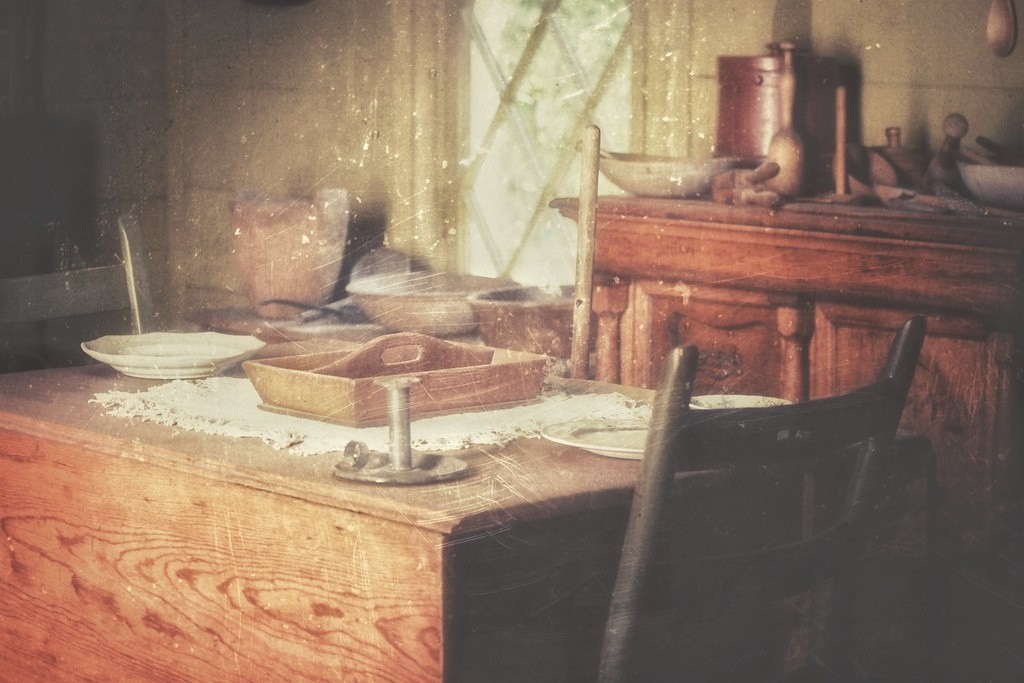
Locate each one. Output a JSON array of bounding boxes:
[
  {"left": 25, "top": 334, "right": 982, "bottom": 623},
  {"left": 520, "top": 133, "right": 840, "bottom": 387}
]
[
  {"left": 80, "top": 331, "right": 267, "bottom": 380},
  {"left": 689, "top": 395, "right": 795, "bottom": 411},
  {"left": 540, "top": 417, "right": 649, "bottom": 460}
]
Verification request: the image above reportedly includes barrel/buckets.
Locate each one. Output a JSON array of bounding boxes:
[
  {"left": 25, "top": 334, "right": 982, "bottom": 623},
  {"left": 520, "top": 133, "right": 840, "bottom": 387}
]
[{"left": 717, "top": 43, "right": 850, "bottom": 197}]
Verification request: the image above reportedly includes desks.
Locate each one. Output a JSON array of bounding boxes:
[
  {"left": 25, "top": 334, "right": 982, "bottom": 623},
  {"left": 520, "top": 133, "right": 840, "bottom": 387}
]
[{"left": 0, "top": 361, "right": 934, "bottom": 683}]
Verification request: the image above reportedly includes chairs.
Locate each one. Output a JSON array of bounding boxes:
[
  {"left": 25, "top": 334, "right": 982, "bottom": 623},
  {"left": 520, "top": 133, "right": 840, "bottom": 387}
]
[
  {"left": 0, "top": 216, "right": 154, "bottom": 373},
  {"left": 600, "top": 317, "right": 926, "bottom": 683}
]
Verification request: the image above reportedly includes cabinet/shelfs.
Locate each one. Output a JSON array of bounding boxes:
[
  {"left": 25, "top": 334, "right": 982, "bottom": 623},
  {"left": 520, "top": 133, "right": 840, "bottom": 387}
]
[{"left": 550, "top": 191, "right": 1024, "bottom": 683}]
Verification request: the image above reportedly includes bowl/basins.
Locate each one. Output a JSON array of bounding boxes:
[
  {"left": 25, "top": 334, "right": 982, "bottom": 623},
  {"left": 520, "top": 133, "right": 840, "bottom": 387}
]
[
  {"left": 344, "top": 270, "right": 522, "bottom": 338},
  {"left": 597, "top": 152, "right": 742, "bottom": 199},
  {"left": 467, "top": 283, "right": 598, "bottom": 357},
  {"left": 957, "top": 160, "right": 1024, "bottom": 210}
]
